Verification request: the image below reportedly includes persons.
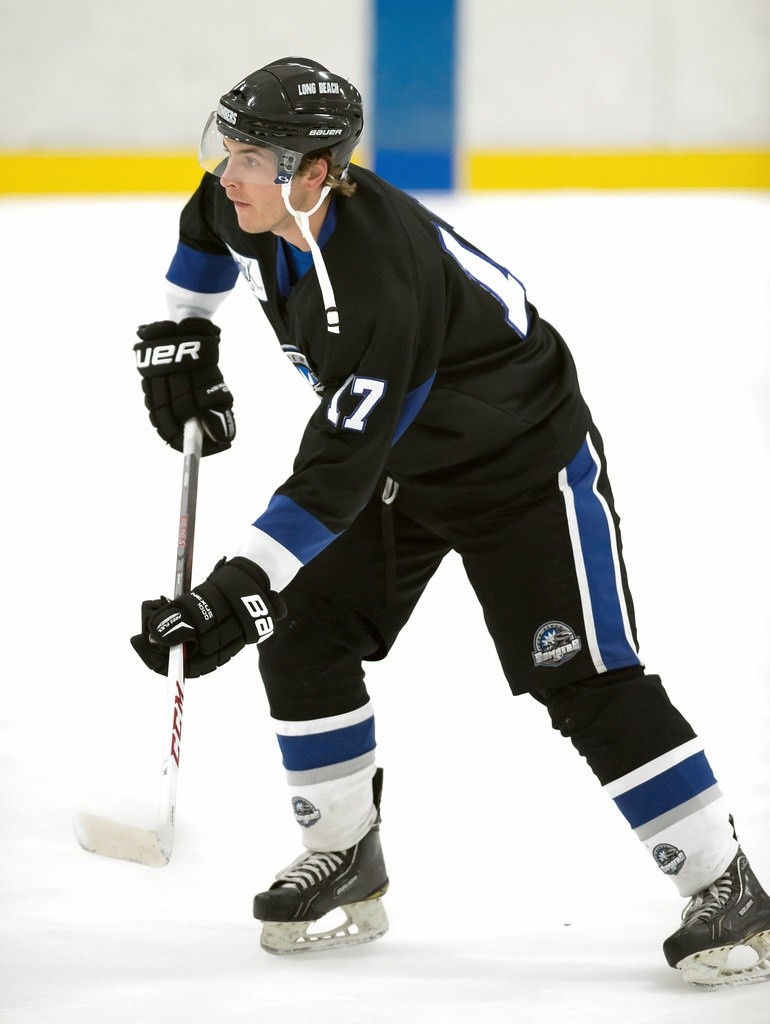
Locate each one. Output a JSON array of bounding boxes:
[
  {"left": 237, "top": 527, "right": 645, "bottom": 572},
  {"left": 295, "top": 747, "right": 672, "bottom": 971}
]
[{"left": 127, "top": 57, "right": 770, "bottom": 990}]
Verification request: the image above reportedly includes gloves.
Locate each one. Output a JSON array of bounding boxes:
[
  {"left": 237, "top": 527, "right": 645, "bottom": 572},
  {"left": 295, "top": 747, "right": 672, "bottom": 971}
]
[
  {"left": 132, "top": 316, "right": 236, "bottom": 458},
  {"left": 130, "top": 555, "right": 287, "bottom": 679}
]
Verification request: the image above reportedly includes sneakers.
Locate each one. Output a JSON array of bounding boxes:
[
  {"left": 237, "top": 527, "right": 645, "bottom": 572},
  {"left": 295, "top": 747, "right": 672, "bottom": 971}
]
[
  {"left": 253, "top": 767, "right": 390, "bottom": 954},
  {"left": 663, "top": 814, "right": 770, "bottom": 989}
]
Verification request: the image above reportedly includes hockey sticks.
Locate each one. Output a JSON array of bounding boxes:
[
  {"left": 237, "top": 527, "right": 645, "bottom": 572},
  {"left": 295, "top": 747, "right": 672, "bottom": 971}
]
[{"left": 70, "top": 418, "right": 202, "bottom": 869}]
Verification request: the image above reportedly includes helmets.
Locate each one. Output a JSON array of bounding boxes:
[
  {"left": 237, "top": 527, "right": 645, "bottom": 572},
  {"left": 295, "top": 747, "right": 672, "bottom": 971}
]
[{"left": 200, "top": 57, "right": 364, "bottom": 183}]
